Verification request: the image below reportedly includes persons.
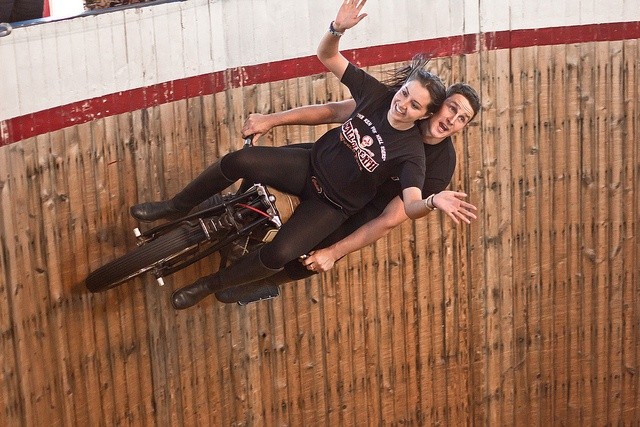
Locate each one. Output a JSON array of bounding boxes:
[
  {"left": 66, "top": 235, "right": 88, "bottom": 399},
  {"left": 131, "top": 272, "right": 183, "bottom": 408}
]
[
  {"left": 131, "top": 0, "right": 477, "bottom": 308},
  {"left": 214, "top": 83, "right": 481, "bottom": 304}
]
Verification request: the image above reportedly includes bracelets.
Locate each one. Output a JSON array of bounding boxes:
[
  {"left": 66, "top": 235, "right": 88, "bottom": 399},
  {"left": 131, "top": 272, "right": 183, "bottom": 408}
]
[
  {"left": 329, "top": 20, "right": 345, "bottom": 38},
  {"left": 431, "top": 196, "right": 437, "bottom": 209},
  {"left": 423, "top": 194, "right": 433, "bottom": 210}
]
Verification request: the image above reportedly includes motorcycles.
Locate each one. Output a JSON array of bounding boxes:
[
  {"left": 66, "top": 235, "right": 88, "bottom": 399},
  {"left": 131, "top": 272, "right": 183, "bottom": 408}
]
[{"left": 86, "top": 134, "right": 312, "bottom": 294}]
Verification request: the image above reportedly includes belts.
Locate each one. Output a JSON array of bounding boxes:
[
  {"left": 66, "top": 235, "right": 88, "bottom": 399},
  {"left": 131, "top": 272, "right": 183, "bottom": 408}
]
[{"left": 310, "top": 175, "right": 344, "bottom": 210}]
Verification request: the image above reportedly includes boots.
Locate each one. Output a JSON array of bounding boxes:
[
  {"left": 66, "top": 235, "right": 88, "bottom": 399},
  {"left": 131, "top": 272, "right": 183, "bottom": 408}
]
[
  {"left": 170, "top": 245, "right": 284, "bottom": 310},
  {"left": 130, "top": 153, "right": 236, "bottom": 223}
]
